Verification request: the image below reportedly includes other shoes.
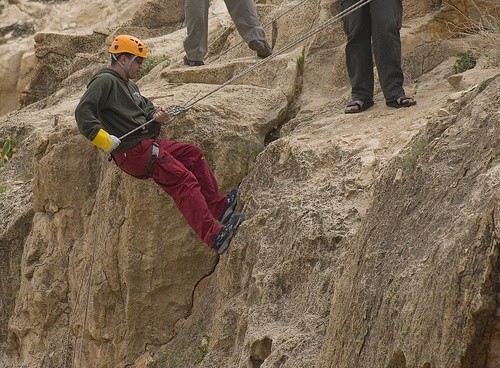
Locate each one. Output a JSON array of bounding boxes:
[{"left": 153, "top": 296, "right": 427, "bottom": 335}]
[
  {"left": 249, "top": 39, "right": 272, "bottom": 58},
  {"left": 183, "top": 56, "right": 203, "bottom": 65}
]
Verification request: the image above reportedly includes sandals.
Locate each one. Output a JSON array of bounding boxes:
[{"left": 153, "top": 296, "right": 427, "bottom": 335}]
[
  {"left": 345, "top": 100, "right": 374, "bottom": 113},
  {"left": 386, "top": 97, "right": 416, "bottom": 107}
]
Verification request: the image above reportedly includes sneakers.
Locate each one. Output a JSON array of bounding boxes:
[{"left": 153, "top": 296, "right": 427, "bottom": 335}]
[
  {"left": 213, "top": 214, "right": 240, "bottom": 255},
  {"left": 218, "top": 189, "right": 239, "bottom": 223}
]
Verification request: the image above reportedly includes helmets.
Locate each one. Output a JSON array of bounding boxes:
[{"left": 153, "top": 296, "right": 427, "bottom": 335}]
[{"left": 109, "top": 34, "right": 147, "bottom": 59}]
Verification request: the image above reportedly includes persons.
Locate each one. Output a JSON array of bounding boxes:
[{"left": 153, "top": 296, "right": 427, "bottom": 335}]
[
  {"left": 183, "top": 0, "right": 272, "bottom": 66},
  {"left": 75, "top": 34, "right": 243, "bottom": 254},
  {"left": 339, "top": 0, "right": 416, "bottom": 114}
]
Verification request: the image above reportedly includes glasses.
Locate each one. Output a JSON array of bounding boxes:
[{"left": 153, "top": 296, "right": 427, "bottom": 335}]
[{"left": 125, "top": 53, "right": 142, "bottom": 64}]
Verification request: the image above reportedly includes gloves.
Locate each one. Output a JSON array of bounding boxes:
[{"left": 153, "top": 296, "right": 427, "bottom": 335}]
[{"left": 102, "top": 134, "right": 121, "bottom": 153}]
